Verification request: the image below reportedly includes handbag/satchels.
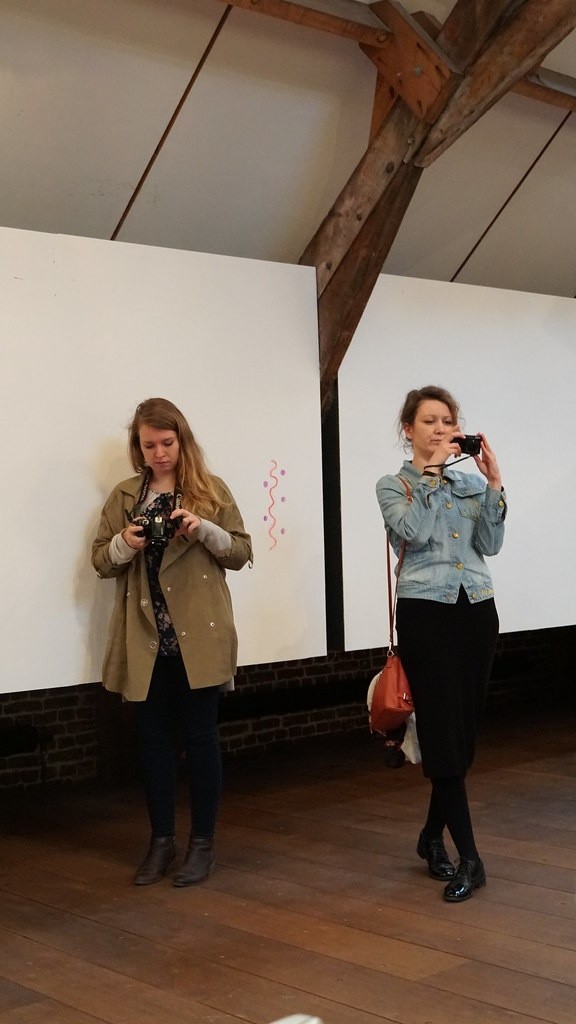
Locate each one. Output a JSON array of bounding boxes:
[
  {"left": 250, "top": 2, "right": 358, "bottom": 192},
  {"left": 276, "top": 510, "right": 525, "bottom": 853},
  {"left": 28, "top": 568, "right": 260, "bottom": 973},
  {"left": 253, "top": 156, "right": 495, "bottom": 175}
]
[{"left": 371, "top": 657, "right": 414, "bottom": 734}]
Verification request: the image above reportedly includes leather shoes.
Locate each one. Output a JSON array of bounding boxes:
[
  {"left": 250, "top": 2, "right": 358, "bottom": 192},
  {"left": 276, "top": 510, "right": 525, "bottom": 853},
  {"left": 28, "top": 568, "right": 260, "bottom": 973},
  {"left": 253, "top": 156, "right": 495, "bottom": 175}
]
[
  {"left": 133, "top": 833, "right": 177, "bottom": 883},
  {"left": 416, "top": 829, "right": 455, "bottom": 881},
  {"left": 443, "top": 856, "right": 486, "bottom": 901},
  {"left": 174, "top": 832, "right": 216, "bottom": 886}
]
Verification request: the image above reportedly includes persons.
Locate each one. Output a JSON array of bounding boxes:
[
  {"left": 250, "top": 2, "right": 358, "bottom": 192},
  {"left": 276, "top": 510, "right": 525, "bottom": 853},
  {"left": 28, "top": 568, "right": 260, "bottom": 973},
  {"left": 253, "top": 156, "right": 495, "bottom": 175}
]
[
  {"left": 376, "top": 386, "right": 507, "bottom": 903},
  {"left": 91, "top": 398, "right": 254, "bottom": 887}
]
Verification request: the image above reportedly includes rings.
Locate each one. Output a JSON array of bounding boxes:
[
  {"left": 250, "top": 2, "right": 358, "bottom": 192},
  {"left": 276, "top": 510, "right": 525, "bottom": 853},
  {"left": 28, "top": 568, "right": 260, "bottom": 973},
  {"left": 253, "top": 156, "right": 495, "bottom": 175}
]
[{"left": 183, "top": 518, "right": 189, "bottom": 525}]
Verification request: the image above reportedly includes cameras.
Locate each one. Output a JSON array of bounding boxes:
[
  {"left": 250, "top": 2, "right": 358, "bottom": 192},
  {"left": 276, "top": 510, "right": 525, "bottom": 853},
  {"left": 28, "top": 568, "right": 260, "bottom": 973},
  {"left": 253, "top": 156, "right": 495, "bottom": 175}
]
[
  {"left": 133, "top": 492, "right": 181, "bottom": 551},
  {"left": 450, "top": 436, "right": 482, "bottom": 456}
]
